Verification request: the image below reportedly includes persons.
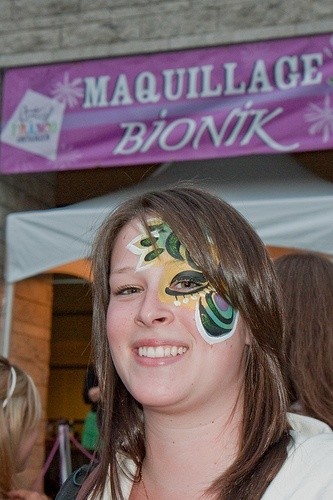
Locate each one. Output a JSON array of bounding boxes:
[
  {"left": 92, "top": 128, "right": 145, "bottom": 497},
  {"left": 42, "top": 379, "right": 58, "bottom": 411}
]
[
  {"left": 274, "top": 253, "right": 333, "bottom": 431},
  {"left": 0, "top": 354, "right": 52, "bottom": 500},
  {"left": 55, "top": 187, "right": 333, "bottom": 500},
  {"left": 82, "top": 362, "right": 101, "bottom": 465}
]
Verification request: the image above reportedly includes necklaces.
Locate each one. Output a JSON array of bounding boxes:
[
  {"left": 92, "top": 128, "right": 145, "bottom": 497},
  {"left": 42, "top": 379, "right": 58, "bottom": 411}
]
[{"left": 139, "top": 471, "right": 149, "bottom": 500}]
